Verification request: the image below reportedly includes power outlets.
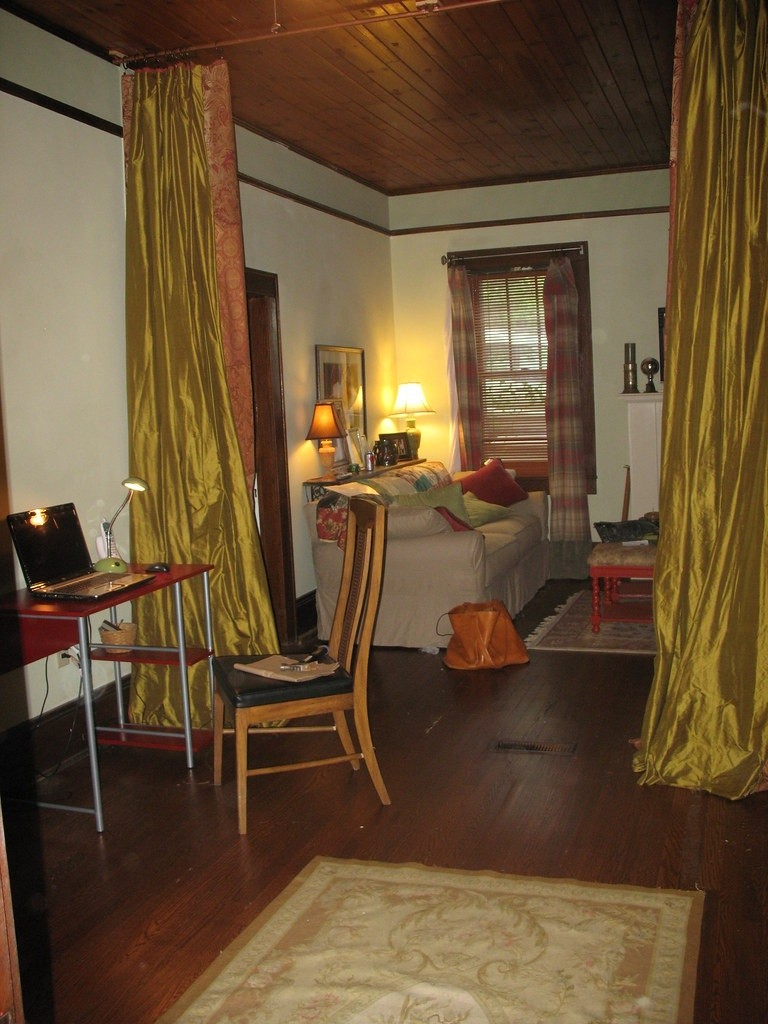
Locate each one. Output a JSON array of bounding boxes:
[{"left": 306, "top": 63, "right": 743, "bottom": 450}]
[{"left": 57, "top": 649, "right": 70, "bottom": 668}]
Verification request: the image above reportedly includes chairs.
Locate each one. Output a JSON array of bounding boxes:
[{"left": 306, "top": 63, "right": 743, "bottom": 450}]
[
  {"left": 211, "top": 496, "right": 387, "bottom": 832},
  {"left": 587, "top": 542, "right": 658, "bottom": 635}
]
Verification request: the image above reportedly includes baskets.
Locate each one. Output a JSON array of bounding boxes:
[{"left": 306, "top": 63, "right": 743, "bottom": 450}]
[{"left": 99, "top": 624, "right": 137, "bottom": 653}]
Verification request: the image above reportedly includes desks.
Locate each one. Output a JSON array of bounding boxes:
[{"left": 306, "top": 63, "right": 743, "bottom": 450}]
[
  {"left": 302, "top": 458, "right": 426, "bottom": 502},
  {"left": 0, "top": 561, "right": 216, "bottom": 831}
]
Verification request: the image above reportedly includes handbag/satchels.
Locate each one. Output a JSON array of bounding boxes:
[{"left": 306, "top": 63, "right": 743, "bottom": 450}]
[
  {"left": 593, "top": 518, "right": 658, "bottom": 544},
  {"left": 436, "top": 600, "right": 530, "bottom": 670}
]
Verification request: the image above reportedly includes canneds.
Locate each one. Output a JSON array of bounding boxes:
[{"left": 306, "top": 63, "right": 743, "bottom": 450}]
[{"left": 365, "top": 452, "right": 374, "bottom": 471}]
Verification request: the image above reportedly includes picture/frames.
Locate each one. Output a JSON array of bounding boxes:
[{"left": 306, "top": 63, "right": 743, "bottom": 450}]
[
  {"left": 379, "top": 432, "right": 414, "bottom": 462},
  {"left": 657, "top": 307, "right": 666, "bottom": 382},
  {"left": 315, "top": 344, "right": 368, "bottom": 449}
]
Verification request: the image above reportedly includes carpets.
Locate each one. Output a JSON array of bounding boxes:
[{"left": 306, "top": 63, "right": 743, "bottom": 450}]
[
  {"left": 523, "top": 589, "right": 656, "bottom": 655},
  {"left": 155, "top": 856, "right": 708, "bottom": 1024}
]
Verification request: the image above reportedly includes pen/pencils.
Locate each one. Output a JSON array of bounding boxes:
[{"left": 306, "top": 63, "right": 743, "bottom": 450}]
[{"left": 117, "top": 618, "right": 124, "bottom": 628}]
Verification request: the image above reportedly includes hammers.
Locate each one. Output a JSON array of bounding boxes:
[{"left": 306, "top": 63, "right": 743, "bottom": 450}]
[{"left": 280, "top": 645, "right": 329, "bottom": 672}]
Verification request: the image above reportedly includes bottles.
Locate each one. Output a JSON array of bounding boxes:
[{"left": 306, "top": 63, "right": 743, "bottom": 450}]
[
  {"left": 366, "top": 452, "right": 374, "bottom": 471},
  {"left": 373, "top": 441, "right": 380, "bottom": 459}
]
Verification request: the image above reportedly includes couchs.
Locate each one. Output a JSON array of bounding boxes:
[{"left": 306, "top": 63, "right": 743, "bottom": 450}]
[{"left": 299, "top": 461, "right": 554, "bottom": 648}]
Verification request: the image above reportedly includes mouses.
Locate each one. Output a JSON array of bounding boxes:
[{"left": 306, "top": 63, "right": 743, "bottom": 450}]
[{"left": 145, "top": 563, "right": 169, "bottom": 573}]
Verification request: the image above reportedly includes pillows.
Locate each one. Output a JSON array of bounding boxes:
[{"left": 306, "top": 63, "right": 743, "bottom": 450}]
[
  {"left": 462, "top": 491, "right": 515, "bottom": 527},
  {"left": 394, "top": 481, "right": 472, "bottom": 527},
  {"left": 457, "top": 458, "right": 529, "bottom": 508}
]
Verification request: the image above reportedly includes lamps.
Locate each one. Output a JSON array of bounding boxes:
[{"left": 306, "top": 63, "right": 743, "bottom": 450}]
[
  {"left": 390, "top": 383, "right": 437, "bottom": 460},
  {"left": 305, "top": 401, "right": 348, "bottom": 482},
  {"left": 93, "top": 477, "right": 149, "bottom": 573}
]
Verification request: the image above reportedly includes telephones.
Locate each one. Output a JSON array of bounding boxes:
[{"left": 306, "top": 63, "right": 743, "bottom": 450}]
[{"left": 95, "top": 517, "right": 123, "bottom": 560}]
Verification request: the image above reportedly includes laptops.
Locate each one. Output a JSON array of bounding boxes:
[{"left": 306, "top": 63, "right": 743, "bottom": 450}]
[{"left": 7, "top": 502, "right": 155, "bottom": 601}]
